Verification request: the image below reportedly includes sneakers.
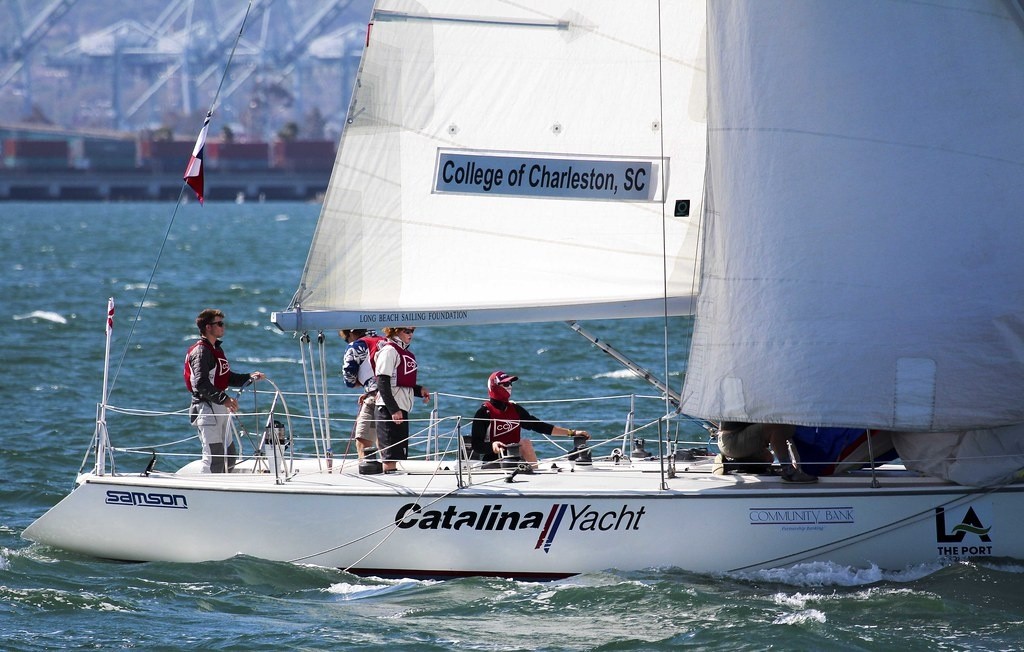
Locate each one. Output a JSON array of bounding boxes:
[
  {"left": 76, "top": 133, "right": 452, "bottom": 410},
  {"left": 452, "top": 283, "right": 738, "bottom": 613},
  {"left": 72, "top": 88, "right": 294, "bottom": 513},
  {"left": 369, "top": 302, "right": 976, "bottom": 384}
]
[
  {"left": 711, "top": 453, "right": 729, "bottom": 476},
  {"left": 781, "top": 467, "right": 819, "bottom": 484}
]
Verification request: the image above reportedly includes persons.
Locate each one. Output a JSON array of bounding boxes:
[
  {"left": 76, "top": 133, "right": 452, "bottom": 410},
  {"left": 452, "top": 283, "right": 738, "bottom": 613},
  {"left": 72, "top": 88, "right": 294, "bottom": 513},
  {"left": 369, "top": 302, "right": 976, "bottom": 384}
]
[
  {"left": 182, "top": 309, "right": 266, "bottom": 473},
  {"left": 339, "top": 329, "right": 387, "bottom": 462},
  {"left": 471, "top": 371, "right": 593, "bottom": 468},
  {"left": 374, "top": 326, "right": 429, "bottom": 473}
]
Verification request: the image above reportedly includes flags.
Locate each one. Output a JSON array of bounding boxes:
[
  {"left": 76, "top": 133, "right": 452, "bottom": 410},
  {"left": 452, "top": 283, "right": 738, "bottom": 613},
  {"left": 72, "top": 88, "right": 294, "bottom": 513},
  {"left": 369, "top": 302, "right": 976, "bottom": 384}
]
[{"left": 183, "top": 111, "right": 210, "bottom": 207}]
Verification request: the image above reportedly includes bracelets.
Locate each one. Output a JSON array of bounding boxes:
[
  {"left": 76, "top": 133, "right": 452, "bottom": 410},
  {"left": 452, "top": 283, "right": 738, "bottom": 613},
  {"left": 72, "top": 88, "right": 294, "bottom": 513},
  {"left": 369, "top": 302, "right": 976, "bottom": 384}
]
[
  {"left": 568, "top": 428, "right": 572, "bottom": 436},
  {"left": 573, "top": 429, "right": 576, "bottom": 436}
]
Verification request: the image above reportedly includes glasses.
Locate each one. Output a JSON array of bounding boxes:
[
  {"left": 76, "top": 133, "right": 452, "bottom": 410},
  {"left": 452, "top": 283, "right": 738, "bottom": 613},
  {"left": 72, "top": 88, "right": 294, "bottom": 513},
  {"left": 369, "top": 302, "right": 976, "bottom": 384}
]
[
  {"left": 210, "top": 321, "right": 223, "bottom": 327},
  {"left": 402, "top": 328, "right": 414, "bottom": 334},
  {"left": 499, "top": 382, "right": 513, "bottom": 387},
  {"left": 344, "top": 334, "right": 350, "bottom": 343}
]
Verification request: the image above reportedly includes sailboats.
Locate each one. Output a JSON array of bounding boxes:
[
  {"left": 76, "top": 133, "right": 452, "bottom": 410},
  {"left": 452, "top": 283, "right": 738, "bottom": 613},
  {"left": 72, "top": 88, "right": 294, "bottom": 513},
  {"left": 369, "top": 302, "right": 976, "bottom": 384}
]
[{"left": 21, "top": 1, "right": 1024, "bottom": 585}]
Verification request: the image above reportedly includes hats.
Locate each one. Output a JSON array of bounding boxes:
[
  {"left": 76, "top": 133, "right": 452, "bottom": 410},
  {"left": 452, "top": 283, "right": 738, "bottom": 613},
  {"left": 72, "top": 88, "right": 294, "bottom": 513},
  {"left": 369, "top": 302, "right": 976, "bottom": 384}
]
[{"left": 494, "top": 372, "right": 518, "bottom": 385}]
